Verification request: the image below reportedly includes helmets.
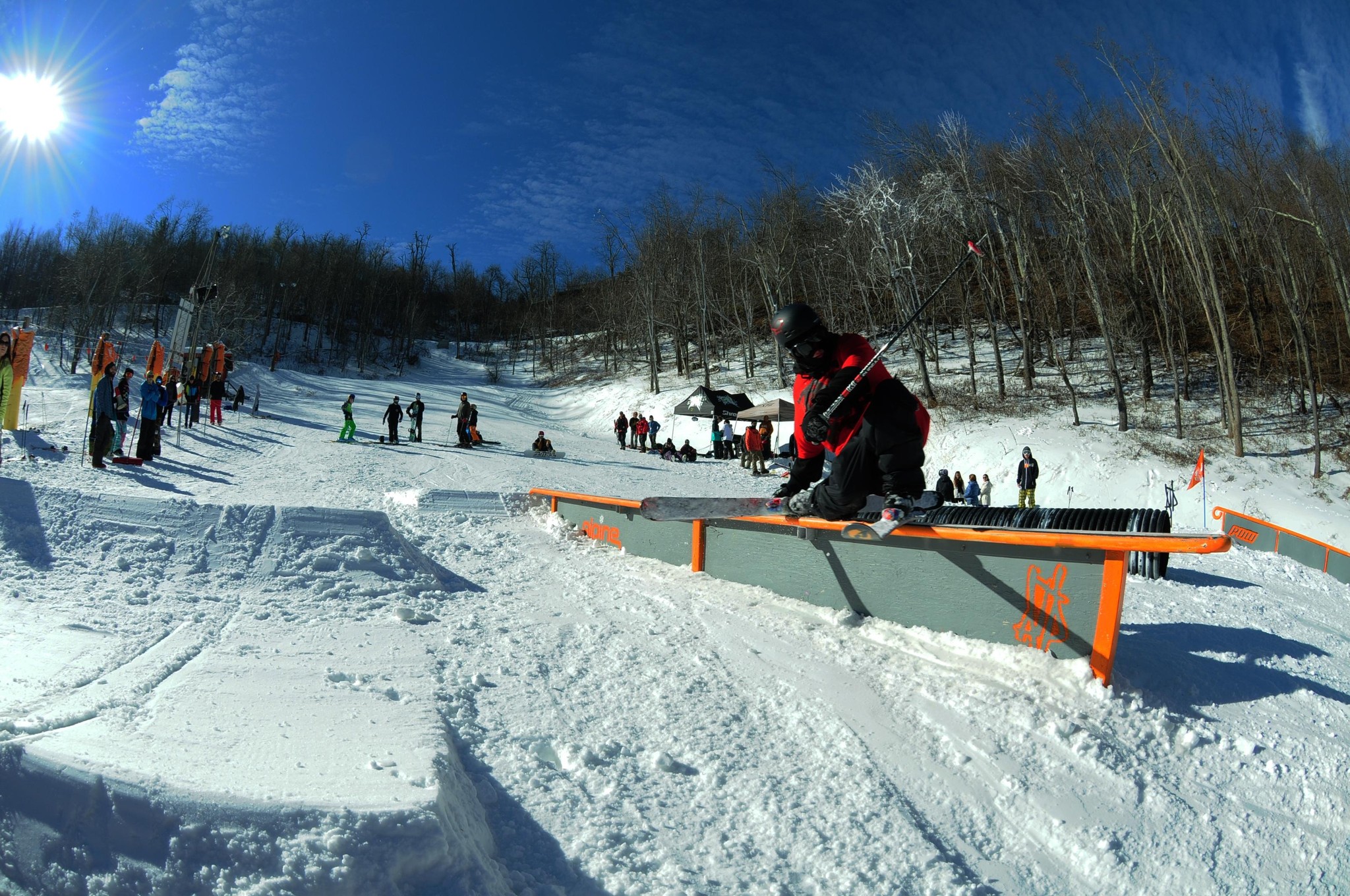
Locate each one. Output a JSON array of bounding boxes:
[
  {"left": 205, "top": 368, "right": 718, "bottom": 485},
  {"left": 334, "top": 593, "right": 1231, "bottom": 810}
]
[
  {"left": 772, "top": 303, "right": 824, "bottom": 362},
  {"left": 538, "top": 431, "right": 545, "bottom": 438},
  {"left": 939, "top": 469, "right": 948, "bottom": 478},
  {"left": 471, "top": 404, "right": 477, "bottom": 409}
]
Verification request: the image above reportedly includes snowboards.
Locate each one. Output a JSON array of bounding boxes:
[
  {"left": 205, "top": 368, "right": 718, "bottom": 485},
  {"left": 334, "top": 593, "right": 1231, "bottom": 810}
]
[
  {"left": 523, "top": 450, "right": 566, "bottom": 460},
  {"left": 408, "top": 403, "right": 419, "bottom": 443},
  {"left": 364, "top": 441, "right": 409, "bottom": 447},
  {"left": 480, "top": 440, "right": 501, "bottom": 445}
]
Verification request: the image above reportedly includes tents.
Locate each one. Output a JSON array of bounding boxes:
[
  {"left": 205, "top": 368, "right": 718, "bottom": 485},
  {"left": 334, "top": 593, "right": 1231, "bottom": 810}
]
[
  {"left": 730, "top": 398, "right": 793, "bottom": 457},
  {"left": 671, "top": 385, "right": 755, "bottom": 453}
]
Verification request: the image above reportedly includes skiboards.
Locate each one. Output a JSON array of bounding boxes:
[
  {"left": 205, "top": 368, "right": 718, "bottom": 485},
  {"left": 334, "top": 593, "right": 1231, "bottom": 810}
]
[
  {"left": 31, "top": 444, "right": 87, "bottom": 452},
  {"left": 438, "top": 442, "right": 473, "bottom": 449},
  {"left": 330, "top": 438, "right": 370, "bottom": 445},
  {"left": 14, "top": 429, "right": 58, "bottom": 434},
  {"left": 640, "top": 491, "right": 944, "bottom": 542},
  {"left": 241, "top": 413, "right": 283, "bottom": 420}
]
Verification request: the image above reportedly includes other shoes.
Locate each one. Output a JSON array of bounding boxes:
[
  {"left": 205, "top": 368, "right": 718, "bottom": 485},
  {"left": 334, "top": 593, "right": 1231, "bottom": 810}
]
[
  {"left": 671, "top": 455, "right": 769, "bottom": 475},
  {"left": 338, "top": 437, "right": 480, "bottom": 448},
  {"left": 92, "top": 463, "right": 106, "bottom": 468},
  {"left": 136, "top": 453, "right": 151, "bottom": 461},
  {"left": 620, "top": 445, "right": 646, "bottom": 453},
  {"left": 152, "top": 446, "right": 160, "bottom": 455},
  {"left": 113, "top": 448, "right": 124, "bottom": 456}
]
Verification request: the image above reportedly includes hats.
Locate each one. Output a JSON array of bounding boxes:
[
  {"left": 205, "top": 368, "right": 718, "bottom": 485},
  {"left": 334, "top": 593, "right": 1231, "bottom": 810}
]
[
  {"left": 346, "top": 393, "right": 467, "bottom": 403},
  {"left": 123, "top": 368, "right": 134, "bottom": 380},
  {"left": 146, "top": 371, "right": 222, "bottom": 381}
]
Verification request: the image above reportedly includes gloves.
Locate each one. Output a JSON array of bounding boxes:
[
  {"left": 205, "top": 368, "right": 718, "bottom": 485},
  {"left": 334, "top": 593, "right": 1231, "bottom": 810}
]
[
  {"left": 802, "top": 398, "right": 835, "bottom": 445},
  {"left": 773, "top": 483, "right": 802, "bottom": 498}
]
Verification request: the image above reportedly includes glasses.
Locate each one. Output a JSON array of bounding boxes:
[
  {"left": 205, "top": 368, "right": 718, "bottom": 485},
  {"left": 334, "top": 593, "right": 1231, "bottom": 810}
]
[
  {"left": 792, "top": 322, "right": 827, "bottom": 358},
  {"left": 1022, "top": 450, "right": 1032, "bottom": 456},
  {"left": 148, "top": 375, "right": 154, "bottom": 378},
  {"left": 462, "top": 396, "right": 466, "bottom": 398},
  {"left": 0, "top": 339, "right": 10, "bottom": 347}
]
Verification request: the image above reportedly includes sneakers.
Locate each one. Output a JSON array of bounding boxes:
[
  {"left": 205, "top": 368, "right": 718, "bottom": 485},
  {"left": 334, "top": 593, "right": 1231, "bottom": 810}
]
[
  {"left": 790, "top": 491, "right": 814, "bottom": 515},
  {"left": 880, "top": 493, "right": 915, "bottom": 522}
]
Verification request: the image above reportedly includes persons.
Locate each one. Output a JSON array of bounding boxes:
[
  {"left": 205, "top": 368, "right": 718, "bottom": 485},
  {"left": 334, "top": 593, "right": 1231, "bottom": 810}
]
[
  {"left": 337, "top": 394, "right": 357, "bottom": 442},
  {"left": 979, "top": 474, "right": 994, "bottom": 508},
  {"left": 771, "top": 303, "right": 931, "bottom": 522},
  {"left": 451, "top": 392, "right": 481, "bottom": 448},
  {"left": 1015, "top": 446, "right": 1040, "bottom": 508},
  {"left": 614, "top": 411, "right": 660, "bottom": 453},
  {"left": 711, "top": 415, "right": 774, "bottom": 476},
  {"left": 953, "top": 471, "right": 965, "bottom": 504},
  {"left": 209, "top": 372, "right": 225, "bottom": 425},
  {"left": 136, "top": 371, "right": 178, "bottom": 461},
  {"left": 935, "top": 469, "right": 954, "bottom": 502},
  {"left": 963, "top": 474, "right": 979, "bottom": 507},
  {"left": 183, "top": 375, "right": 198, "bottom": 429},
  {"left": 0, "top": 333, "right": 13, "bottom": 467},
  {"left": 532, "top": 431, "right": 556, "bottom": 452},
  {"left": 87, "top": 361, "right": 133, "bottom": 469},
  {"left": 662, "top": 438, "right": 697, "bottom": 462},
  {"left": 382, "top": 393, "right": 424, "bottom": 444}
]
